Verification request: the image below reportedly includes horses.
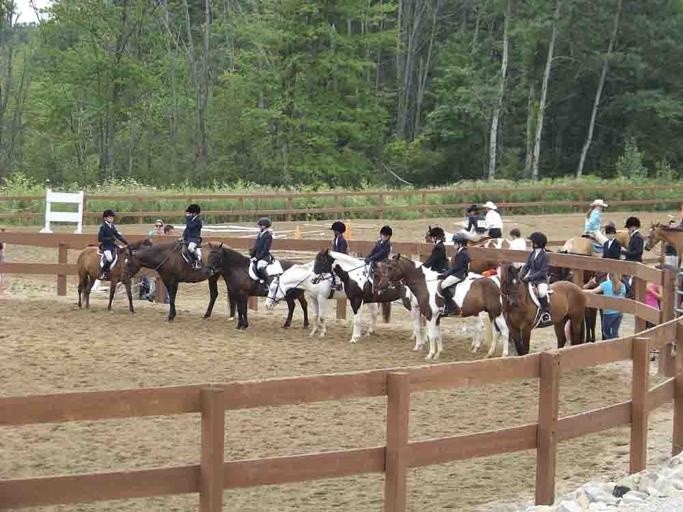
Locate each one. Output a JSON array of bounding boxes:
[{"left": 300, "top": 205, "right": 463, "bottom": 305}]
[
  {"left": 76, "top": 237, "right": 154, "bottom": 315},
  {"left": 206, "top": 241, "right": 308, "bottom": 332},
  {"left": 119, "top": 241, "right": 236, "bottom": 322},
  {"left": 263, "top": 260, "right": 378, "bottom": 338},
  {"left": 363, "top": 219, "right": 683, "bottom": 361},
  {"left": 313, "top": 248, "right": 415, "bottom": 343}
]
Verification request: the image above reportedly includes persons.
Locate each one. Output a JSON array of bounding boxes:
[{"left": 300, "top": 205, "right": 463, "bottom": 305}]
[
  {"left": 164, "top": 224, "right": 175, "bottom": 237},
  {"left": 420, "top": 227, "right": 449, "bottom": 275},
  {"left": 144, "top": 217, "right": 163, "bottom": 303},
  {"left": 249, "top": 214, "right": 272, "bottom": 293},
  {"left": 183, "top": 203, "right": 204, "bottom": 272},
  {"left": 327, "top": 221, "right": 347, "bottom": 292},
  {"left": 361, "top": 226, "right": 392, "bottom": 292},
  {"left": 435, "top": 231, "right": 469, "bottom": 315},
  {"left": 95, "top": 209, "right": 130, "bottom": 279},
  {"left": 464, "top": 195, "right": 682, "bottom": 343}
]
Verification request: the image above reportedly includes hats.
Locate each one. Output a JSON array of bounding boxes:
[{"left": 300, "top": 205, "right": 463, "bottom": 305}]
[
  {"left": 466, "top": 202, "right": 497, "bottom": 211},
  {"left": 590, "top": 200, "right": 607, "bottom": 208}
]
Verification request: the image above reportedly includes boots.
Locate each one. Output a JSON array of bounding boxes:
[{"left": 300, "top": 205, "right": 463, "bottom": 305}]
[
  {"left": 442, "top": 289, "right": 457, "bottom": 315},
  {"left": 189, "top": 252, "right": 198, "bottom": 267},
  {"left": 538, "top": 295, "right": 549, "bottom": 320},
  {"left": 103, "top": 260, "right": 112, "bottom": 275}
]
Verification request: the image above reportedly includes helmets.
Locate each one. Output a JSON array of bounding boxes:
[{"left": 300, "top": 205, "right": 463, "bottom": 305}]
[
  {"left": 103, "top": 210, "right": 115, "bottom": 217},
  {"left": 380, "top": 226, "right": 392, "bottom": 236},
  {"left": 427, "top": 228, "right": 469, "bottom": 241},
  {"left": 605, "top": 217, "right": 639, "bottom": 232},
  {"left": 258, "top": 218, "right": 271, "bottom": 226},
  {"left": 186, "top": 204, "right": 201, "bottom": 212},
  {"left": 329, "top": 222, "right": 346, "bottom": 232},
  {"left": 527, "top": 232, "right": 547, "bottom": 243}
]
